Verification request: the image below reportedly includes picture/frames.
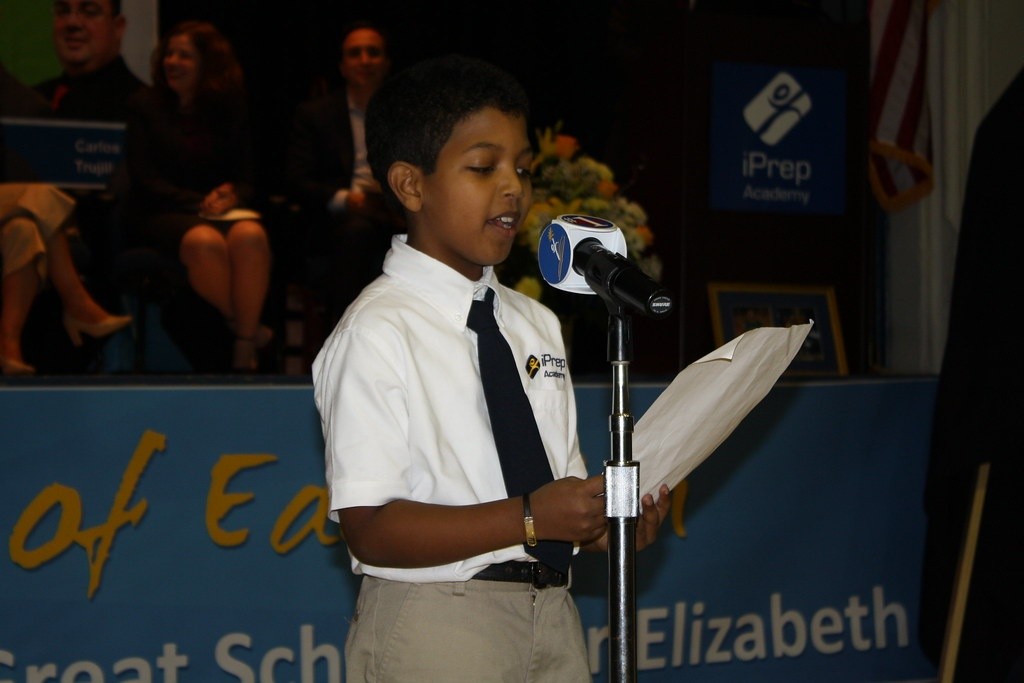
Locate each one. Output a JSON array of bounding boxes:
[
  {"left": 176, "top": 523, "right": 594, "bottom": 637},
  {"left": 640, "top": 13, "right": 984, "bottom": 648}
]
[{"left": 706, "top": 282, "right": 850, "bottom": 382}]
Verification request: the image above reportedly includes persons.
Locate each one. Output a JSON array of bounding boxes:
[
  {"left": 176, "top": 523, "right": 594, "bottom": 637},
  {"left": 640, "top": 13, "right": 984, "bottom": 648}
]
[
  {"left": 125, "top": 22, "right": 273, "bottom": 374},
  {"left": 0, "top": 183, "right": 132, "bottom": 374},
  {"left": 311, "top": 57, "right": 670, "bottom": 683},
  {"left": 294, "top": 28, "right": 407, "bottom": 338},
  {"left": 32, "top": 0, "right": 150, "bottom": 123}
]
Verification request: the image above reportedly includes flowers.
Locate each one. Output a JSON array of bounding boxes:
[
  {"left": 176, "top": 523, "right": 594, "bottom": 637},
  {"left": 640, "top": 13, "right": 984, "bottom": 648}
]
[{"left": 508, "top": 117, "right": 664, "bottom": 309}]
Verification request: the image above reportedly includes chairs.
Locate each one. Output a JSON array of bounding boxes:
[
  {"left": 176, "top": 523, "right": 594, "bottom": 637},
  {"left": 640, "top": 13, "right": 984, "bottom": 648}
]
[{"left": 85, "top": 192, "right": 302, "bottom": 378}]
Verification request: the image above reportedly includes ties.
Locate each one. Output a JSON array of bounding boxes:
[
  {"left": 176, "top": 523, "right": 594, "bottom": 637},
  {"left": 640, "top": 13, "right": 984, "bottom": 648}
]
[
  {"left": 467, "top": 286, "right": 576, "bottom": 567},
  {"left": 45, "top": 85, "right": 70, "bottom": 109}
]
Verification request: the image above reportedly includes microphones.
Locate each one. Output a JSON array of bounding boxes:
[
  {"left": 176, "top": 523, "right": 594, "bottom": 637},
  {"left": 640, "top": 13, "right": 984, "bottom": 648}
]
[{"left": 537, "top": 214, "right": 673, "bottom": 317}]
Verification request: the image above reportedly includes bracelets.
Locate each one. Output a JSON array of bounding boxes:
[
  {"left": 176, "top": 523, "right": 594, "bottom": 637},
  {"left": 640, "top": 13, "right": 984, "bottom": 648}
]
[{"left": 522, "top": 494, "right": 537, "bottom": 548}]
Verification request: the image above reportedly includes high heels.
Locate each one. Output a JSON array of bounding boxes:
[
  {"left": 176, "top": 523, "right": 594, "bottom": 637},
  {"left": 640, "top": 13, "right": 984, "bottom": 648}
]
[
  {"left": 230, "top": 318, "right": 276, "bottom": 348},
  {"left": 230, "top": 335, "right": 261, "bottom": 377},
  {"left": 62, "top": 310, "right": 132, "bottom": 347},
  {"left": 1, "top": 354, "right": 38, "bottom": 378}
]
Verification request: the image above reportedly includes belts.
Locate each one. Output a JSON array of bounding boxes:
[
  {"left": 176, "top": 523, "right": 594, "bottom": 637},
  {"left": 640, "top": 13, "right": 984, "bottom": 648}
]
[{"left": 475, "top": 559, "right": 572, "bottom": 592}]
